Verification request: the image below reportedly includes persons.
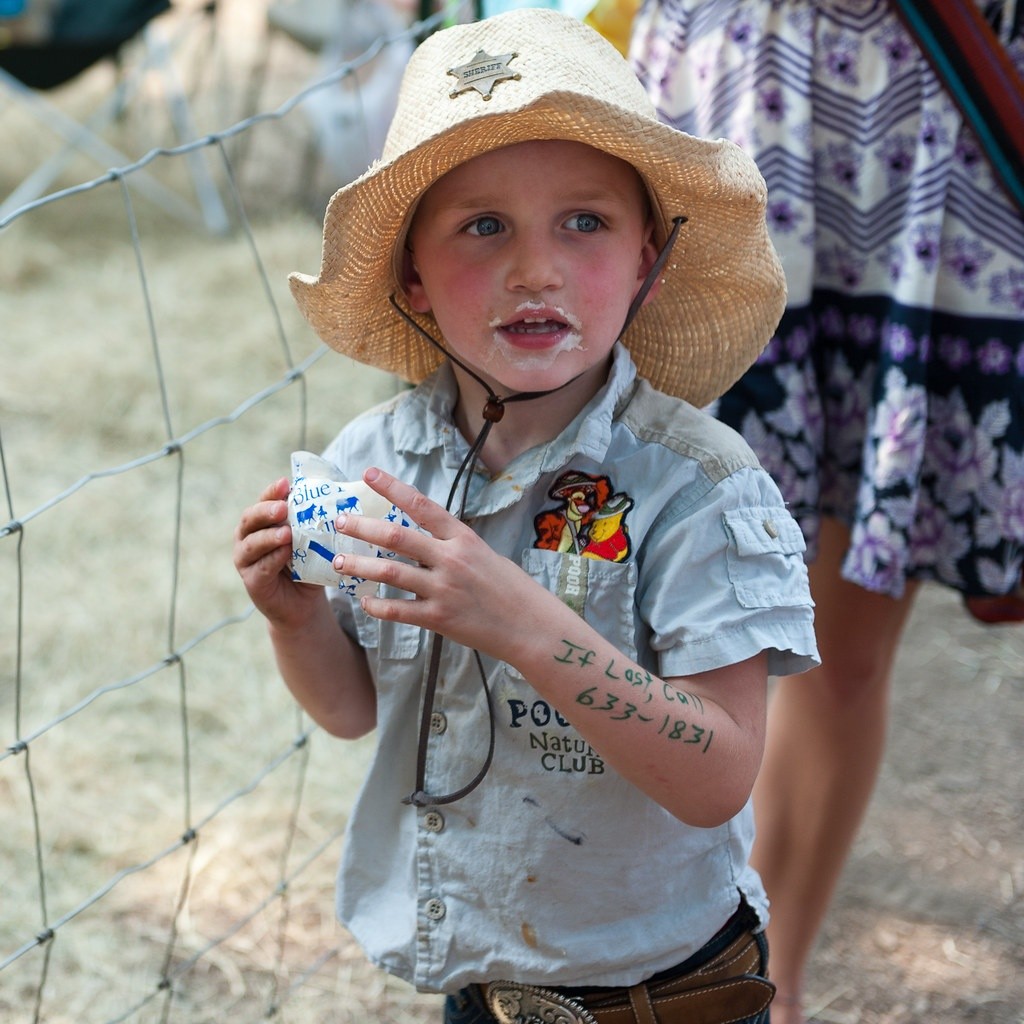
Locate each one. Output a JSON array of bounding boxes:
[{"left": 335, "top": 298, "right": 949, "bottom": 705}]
[
  {"left": 230, "top": 8, "right": 823, "bottom": 1024},
  {"left": 628, "top": 0, "right": 1024, "bottom": 1024}
]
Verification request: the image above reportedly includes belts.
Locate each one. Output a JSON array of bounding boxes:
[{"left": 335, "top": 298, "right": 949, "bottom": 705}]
[{"left": 480, "top": 930, "right": 777, "bottom": 1024}]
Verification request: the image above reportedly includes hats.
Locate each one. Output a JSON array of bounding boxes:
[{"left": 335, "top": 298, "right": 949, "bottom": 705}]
[{"left": 287, "top": 7, "right": 788, "bottom": 410}]
